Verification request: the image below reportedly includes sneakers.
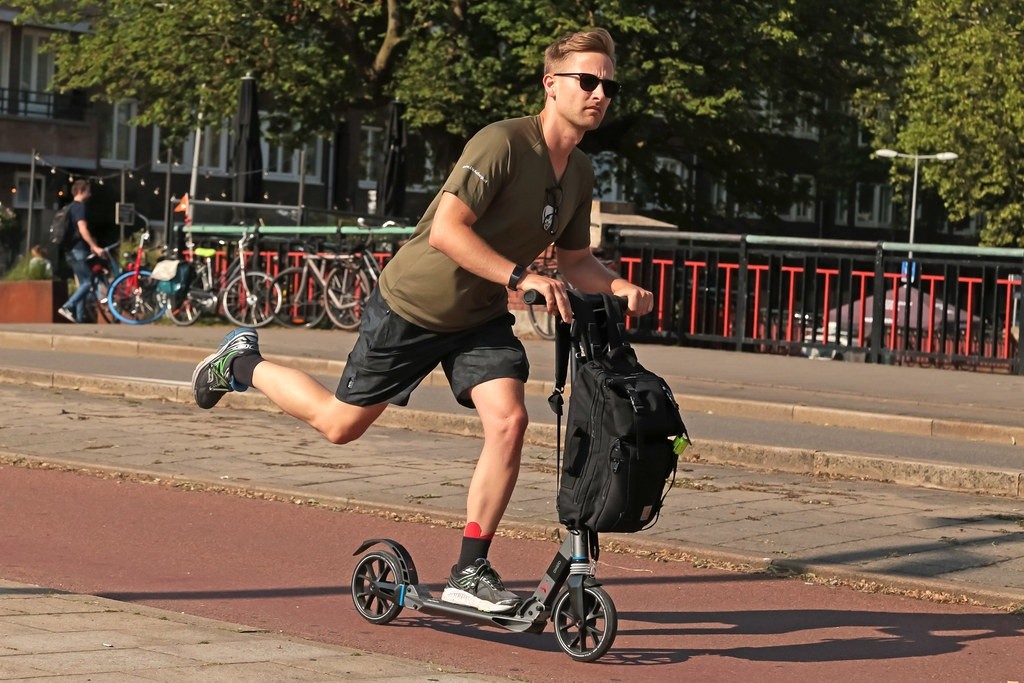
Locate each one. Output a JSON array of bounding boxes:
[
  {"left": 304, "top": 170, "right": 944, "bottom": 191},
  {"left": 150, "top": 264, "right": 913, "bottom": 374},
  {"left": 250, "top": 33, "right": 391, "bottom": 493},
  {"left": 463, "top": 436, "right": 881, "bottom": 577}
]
[
  {"left": 441, "top": 559, "right": 523, "bottom": 613},
  {"left": 58, "top": 307, "right": 77, "bottom": 323},
  {"left": 191, "top": 328, "right": 261, "bottom": 410}
]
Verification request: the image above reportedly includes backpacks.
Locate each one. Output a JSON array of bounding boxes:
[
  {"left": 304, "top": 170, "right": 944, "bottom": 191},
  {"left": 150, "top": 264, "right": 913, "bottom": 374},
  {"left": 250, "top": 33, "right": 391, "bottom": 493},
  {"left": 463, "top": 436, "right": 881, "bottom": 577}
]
[
  {"left": 49, "top": 201, "right": 78, "bottom": 245},
  {"left": 548, "top": 292, "right": 692, "bottom": 536}
]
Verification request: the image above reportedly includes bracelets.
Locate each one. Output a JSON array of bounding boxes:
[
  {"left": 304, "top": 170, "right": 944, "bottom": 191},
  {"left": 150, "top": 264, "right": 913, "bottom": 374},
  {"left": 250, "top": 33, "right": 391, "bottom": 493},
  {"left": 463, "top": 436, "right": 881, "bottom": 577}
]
[{"left": 507, "top": 263, "right": 522, "bottom": 295}]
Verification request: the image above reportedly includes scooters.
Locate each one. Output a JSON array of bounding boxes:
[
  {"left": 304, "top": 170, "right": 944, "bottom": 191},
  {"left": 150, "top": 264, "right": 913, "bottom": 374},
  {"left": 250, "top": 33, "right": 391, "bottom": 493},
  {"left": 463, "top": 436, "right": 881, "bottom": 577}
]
[{"left": 352, "top": 289, "right": 644, "bottom": 663}]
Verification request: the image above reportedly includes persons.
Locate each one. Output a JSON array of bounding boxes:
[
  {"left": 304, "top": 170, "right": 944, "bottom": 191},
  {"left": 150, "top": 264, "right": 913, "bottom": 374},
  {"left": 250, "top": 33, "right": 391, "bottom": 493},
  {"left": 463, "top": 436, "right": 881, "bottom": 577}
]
[
  {"left": 190, "top": 27, "right": 654, "bottom": 613},
  {"left": 28, "top": 180, "right": 105, "bottom": 324}
]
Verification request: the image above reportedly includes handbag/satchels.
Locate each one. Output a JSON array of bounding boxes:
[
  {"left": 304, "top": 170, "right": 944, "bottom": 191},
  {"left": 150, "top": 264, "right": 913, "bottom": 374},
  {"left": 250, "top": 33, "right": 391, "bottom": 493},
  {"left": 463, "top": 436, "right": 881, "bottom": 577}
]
[{"left": 150, "top": 259, "right": 191, "bottom": 296}]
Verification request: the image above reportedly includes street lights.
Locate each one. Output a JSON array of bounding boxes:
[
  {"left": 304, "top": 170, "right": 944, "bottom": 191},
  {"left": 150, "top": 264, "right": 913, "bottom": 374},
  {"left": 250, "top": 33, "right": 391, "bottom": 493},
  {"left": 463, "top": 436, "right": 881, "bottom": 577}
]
[{"left": 875, "top": 148, "right": 960, "bottom": 256}]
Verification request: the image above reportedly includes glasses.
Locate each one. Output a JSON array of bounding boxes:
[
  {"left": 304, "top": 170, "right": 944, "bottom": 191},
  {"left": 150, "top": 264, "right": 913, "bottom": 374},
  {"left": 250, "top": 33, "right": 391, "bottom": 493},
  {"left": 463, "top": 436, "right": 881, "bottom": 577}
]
[
  {"left": 543, "top": 187, "right": 562, "bottom": 235},
  {"left": 553, "top": 72, "right": 622, "bottom": 98}
]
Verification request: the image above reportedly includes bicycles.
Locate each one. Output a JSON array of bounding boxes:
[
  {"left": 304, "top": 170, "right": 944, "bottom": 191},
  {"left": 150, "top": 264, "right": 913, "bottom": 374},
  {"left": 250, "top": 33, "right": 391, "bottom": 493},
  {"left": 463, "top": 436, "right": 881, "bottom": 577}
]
[{"left": 83, "top": 212, "right": 413, "bottom": 328}]
